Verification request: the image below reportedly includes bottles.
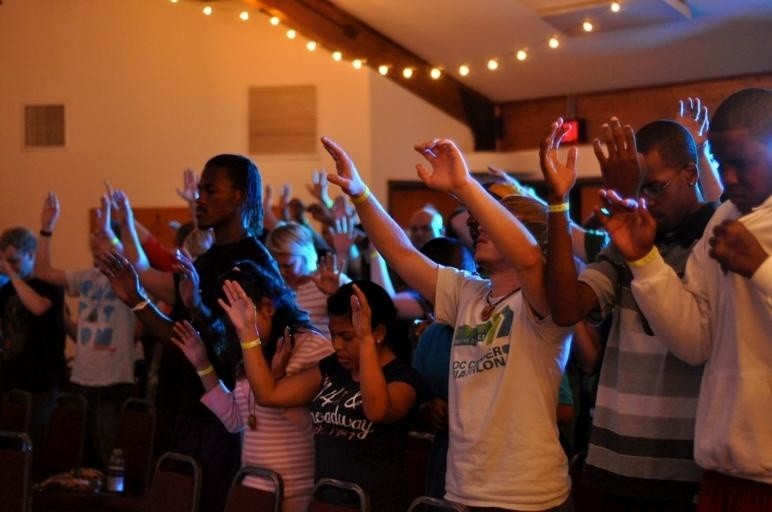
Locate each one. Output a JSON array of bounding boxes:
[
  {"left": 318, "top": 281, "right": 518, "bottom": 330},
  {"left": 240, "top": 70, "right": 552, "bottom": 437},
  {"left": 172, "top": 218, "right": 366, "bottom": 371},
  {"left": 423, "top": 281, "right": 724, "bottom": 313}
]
[{"left": 105, "top": 449, "right": 125, "bottom": 492}]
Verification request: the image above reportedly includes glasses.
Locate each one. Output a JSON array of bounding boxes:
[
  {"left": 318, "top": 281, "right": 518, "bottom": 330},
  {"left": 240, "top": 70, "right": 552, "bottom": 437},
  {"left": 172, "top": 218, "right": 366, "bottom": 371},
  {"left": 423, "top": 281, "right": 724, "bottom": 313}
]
[{"left": 643, "top": 165, "right": 687, "bottom": 198}]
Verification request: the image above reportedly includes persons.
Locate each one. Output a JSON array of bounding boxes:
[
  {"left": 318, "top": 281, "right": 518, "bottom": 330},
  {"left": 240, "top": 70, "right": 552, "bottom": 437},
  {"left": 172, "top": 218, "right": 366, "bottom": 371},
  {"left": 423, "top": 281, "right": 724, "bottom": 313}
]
[{"left": 1, "top": 85, "right": 772, "bottom": 511}]
